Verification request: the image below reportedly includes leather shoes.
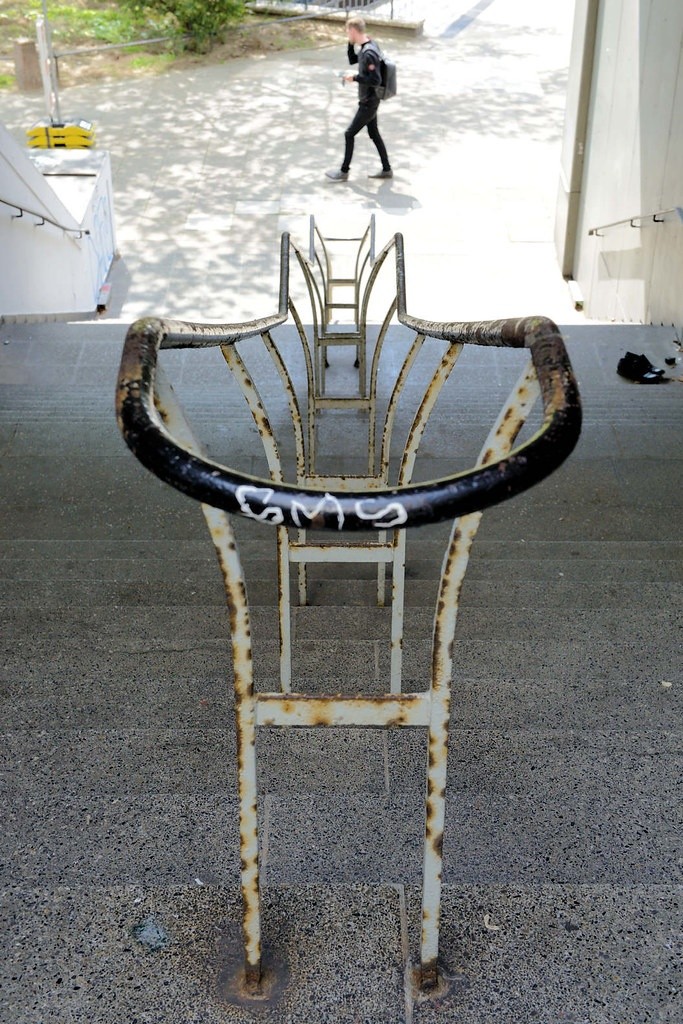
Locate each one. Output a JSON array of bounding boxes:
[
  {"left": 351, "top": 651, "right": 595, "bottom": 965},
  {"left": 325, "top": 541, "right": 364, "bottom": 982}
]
[
  {"left": 624, "top": 351, "right": 665, "bottom": 376},
  {"left": 616, "top": 357, "right": 661, "bottom": 384}
]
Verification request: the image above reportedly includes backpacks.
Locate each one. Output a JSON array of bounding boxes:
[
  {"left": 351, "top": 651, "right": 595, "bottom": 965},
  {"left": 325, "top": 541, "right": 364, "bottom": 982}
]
[{"left": 361, "top": 40, "right": 397, "bottom": 100}]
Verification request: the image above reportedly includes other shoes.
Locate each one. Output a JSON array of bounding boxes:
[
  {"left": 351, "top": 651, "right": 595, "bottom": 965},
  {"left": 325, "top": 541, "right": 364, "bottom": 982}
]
[
  {"left": 325, "top": 169, "right": 349, "bottom": 180},
  {"left": 367, "top": 169, "right": 393, "bottom": 179}
]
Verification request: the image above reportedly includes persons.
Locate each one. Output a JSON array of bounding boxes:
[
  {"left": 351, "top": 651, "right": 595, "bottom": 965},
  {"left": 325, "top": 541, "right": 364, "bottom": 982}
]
[{"left": 325, "top": 17, "right": 393, "bottom": 180}]
